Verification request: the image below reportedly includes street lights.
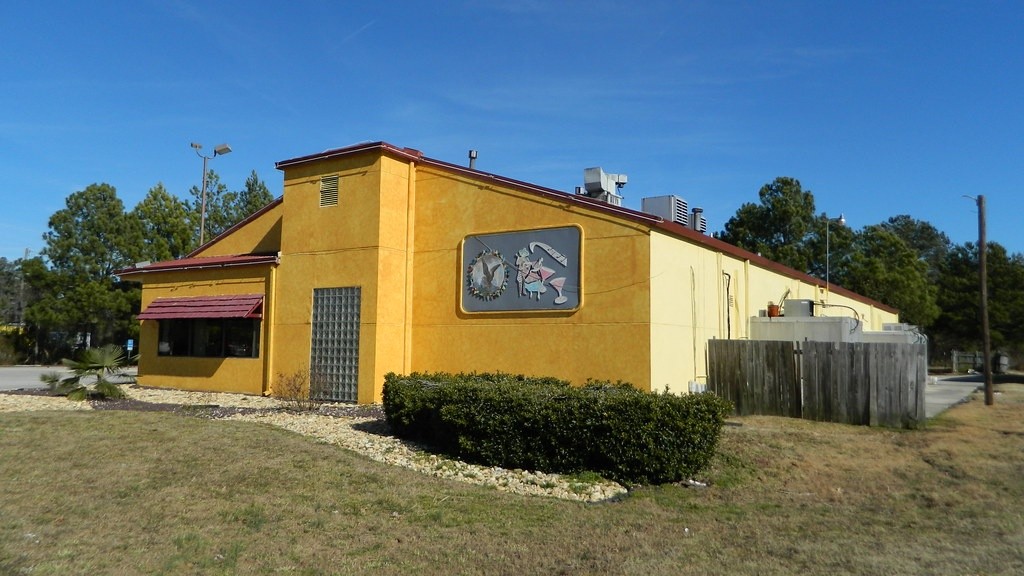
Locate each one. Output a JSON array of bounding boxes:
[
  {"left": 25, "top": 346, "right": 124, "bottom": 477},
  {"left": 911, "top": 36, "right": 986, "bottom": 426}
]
[
  {"left": 189, "top": 143, "right": 232, "bottom": 247},
  {"left": 825, "top": 213, "right": 846, "bottom": 304}
]
